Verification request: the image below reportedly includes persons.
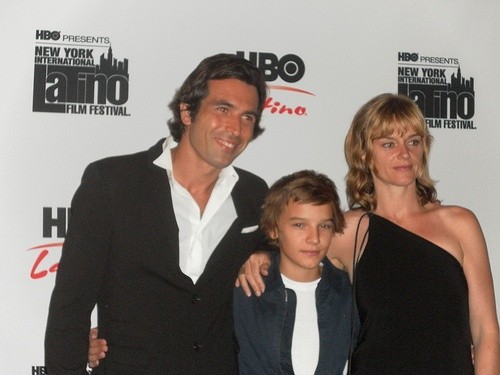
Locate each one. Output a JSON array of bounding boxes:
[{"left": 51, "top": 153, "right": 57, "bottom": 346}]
[
  {"left": 234, "top": 93, "right": 500, "bottom": 375},
  {"left": 86, "top": 168, "right": 476, "bottom": 375},
  {"left": 44, "top": 54, "right": 289, "bottom": 375}
]
